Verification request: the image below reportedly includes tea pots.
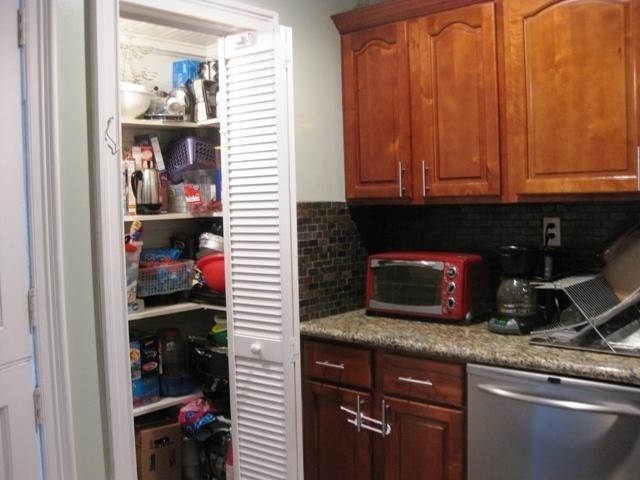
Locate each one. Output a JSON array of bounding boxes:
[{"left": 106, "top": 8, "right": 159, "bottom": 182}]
[{"left": 131, "top": 169, "right": 164, "bottom": 214}]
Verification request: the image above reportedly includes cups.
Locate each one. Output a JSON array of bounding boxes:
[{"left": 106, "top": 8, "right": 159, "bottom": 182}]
[
  {"left": 187, "top": 76, "right": 211, "bottom": 122},
  {"left": 183, "top": 169, "right": 216, "bottom": 215}
]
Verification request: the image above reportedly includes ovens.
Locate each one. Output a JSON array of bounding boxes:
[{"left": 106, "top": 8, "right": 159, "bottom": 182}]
[{"left": 466, "top": 361, "right": 639, "bottom": 478}]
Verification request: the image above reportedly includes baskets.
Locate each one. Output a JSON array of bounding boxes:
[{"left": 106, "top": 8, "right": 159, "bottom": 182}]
[
  {"left": 165, "top": 136, "right": 215, "bottom": 183},
  {"left": 138, "top": 258, "right": 194, "bottom": 297}
]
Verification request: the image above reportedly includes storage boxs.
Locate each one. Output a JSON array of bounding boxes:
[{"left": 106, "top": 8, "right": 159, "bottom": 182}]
[{"left": 135, "top": 422, "right": 181, "bottom": 480}]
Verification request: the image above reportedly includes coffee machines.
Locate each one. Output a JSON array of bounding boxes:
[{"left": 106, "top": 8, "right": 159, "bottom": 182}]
[{"left": 490, "top": 243, "right": 558, "bottom": 333}]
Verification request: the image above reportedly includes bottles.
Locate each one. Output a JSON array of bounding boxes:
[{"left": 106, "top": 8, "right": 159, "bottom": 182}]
[{"left": 156, "top": 328, "right": 182, "bottom": 376}]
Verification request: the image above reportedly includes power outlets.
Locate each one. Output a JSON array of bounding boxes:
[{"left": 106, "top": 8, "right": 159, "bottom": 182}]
[{"left": 543, "top": 217, "right": 561, "bottom": 247}]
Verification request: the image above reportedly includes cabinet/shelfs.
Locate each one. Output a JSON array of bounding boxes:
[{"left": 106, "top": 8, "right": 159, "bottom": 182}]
[
  {"left": 329, "top": 0, "right": 508, "bottom": 208},
  {"left": 300, "top": 336, "right": 467, "bottom": 480},
  {"left": 121, "top": 115, "right": 221, "bottom": 418},
  {"left": 502, "top": 0, "right": 640, "bottom": 205}
]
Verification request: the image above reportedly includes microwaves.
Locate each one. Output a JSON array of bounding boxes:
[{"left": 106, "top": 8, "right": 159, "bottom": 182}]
[{"left": 365, "top": 248, "right": 484, "bottom": 323}]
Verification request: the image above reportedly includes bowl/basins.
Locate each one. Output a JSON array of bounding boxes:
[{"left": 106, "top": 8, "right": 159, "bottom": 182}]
[
  {"left": 152, "top": 88, "right": 188, "bottom": 117},
  {"left": 196, "top": 253, "right": 225, "bottom": 293},
  {"left": 120, "top": 80, "right": 153, "bottom": 118}
]
